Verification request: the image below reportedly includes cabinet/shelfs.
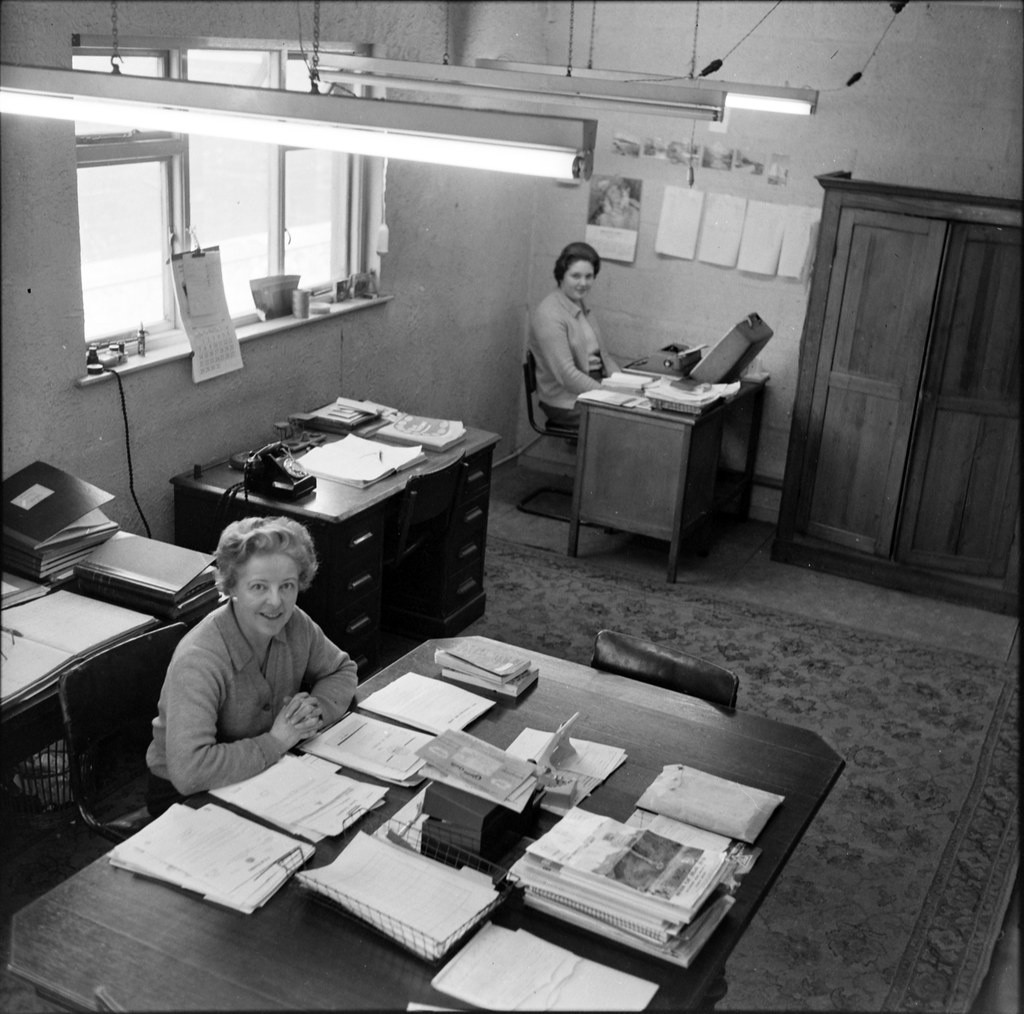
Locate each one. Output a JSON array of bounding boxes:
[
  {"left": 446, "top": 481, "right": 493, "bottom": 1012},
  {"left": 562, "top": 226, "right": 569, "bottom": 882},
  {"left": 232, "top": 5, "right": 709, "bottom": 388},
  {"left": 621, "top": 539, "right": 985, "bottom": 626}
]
[{"left": 770, "top": 169, "right": 1024, "bottom": 618}]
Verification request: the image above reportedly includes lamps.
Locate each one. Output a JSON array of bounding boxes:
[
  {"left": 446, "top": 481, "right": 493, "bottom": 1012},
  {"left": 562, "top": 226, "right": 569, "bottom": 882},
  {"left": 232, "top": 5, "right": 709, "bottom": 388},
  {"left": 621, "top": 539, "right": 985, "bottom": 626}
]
[
  {"left": 477, "top": 0, "right": 820, "bottom": 119},
  {"left": 308, "top": 0, "right": 728, "bottom": 122},
  {"left": 0, "top": 0, "right": 598, "bottom": 182}
]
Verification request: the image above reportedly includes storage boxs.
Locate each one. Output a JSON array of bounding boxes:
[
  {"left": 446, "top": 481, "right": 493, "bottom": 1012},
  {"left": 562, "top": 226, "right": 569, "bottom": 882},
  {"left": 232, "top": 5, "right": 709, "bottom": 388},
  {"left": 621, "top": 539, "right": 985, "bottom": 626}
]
[{"left": 420, "top": 780, "right": 547, "bottom": 871}]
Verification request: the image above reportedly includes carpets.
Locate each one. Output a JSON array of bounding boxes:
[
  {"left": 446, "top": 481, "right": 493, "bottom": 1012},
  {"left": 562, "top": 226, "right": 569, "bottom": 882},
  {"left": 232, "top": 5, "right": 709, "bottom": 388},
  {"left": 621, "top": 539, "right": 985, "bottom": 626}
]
[{"left": 0, "top": 535, "right": 1023, "bottom": 1014}]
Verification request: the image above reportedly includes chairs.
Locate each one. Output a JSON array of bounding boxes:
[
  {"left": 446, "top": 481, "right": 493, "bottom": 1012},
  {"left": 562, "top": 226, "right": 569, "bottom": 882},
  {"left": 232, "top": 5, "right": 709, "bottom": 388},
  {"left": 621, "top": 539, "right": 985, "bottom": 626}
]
[
  {"left": 58, "top": 622, "right": 189, "bottom": 847},
  {"left": 587, "top": 629, "right": 741, "bottom": 712},
  {"left": 517, "top": 351, "right": 599, "bottom": 527}
]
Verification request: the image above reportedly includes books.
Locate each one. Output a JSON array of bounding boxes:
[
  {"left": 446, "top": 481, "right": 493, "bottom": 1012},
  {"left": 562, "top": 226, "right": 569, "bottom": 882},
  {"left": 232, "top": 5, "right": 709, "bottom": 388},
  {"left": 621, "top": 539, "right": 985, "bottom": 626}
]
[
  {"left": 296, "top": 396, "right": 468, "bottom": 489},
  {"left": 508, "top": 807, "right": 737, "bottom": 967},
  {"left": 577, "top": 370, "right": 725, "bottom": 416},
  {"left": 0, "top": 461, "right": 220, "bottom": 709},
  {"left": 434, "top": 637, "right": 542, "bottom": 699}
]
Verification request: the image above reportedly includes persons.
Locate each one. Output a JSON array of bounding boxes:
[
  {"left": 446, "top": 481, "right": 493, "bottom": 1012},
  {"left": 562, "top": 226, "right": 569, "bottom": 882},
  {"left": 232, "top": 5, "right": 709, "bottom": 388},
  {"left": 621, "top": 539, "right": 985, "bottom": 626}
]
[
  {"left": 532, "top": 242, "right": 620, "bottom": 429},
  {"left": 597, "top": 180, "right": 639, "bottom": 229},
  {"left": 147, "top": 516, "right": 359, "bottom": 818}
]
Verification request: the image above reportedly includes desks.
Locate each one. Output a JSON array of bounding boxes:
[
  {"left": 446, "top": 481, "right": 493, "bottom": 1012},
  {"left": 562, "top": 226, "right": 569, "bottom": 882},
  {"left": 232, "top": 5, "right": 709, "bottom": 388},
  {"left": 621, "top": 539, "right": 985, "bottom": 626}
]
[
  {"left": 169, "top": 425, "right": 503, "bottom": 686},
  {"left": 7, "top": 635, "right": 846, "bottom": 1014},
  {"left": 568, "top": 360, "right": 767, "bottom": 583}
]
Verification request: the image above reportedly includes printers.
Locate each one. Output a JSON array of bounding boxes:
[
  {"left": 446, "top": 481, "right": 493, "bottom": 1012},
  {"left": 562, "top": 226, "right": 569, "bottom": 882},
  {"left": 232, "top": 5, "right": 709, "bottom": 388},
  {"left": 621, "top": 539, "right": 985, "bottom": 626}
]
[{"left": 627, "top": 341, "right": 701, "bottom": 377}]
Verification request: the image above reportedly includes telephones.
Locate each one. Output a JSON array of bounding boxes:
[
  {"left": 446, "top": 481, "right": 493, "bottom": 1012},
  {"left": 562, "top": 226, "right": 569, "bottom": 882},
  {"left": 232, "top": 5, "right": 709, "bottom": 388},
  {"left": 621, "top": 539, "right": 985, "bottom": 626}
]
[{"left": 242, "top": 441, "right": 317, "bottom": 503}]
